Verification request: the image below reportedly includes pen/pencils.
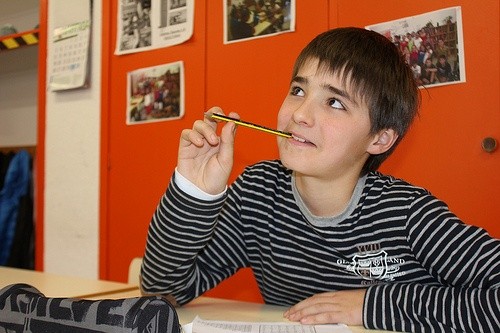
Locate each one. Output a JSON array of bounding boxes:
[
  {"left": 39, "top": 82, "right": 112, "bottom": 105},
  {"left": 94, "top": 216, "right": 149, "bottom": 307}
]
[{"left": 204, "top": 112, "right": 292, "bottom": 138}]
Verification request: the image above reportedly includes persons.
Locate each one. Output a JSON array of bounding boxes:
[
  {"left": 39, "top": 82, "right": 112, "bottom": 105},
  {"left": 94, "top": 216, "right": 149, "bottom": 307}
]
[
  {"left": 138, "top": 27, "right": 500, "bottom": 332},
  {"left": 227, "top": 1, "right": 292, "bottom": 34},
  {"left": 126, "top": 74, "right": 183, "bottom": 117},
  {"left": 368, "top": 24, "right": 455, "bottom": 86}
]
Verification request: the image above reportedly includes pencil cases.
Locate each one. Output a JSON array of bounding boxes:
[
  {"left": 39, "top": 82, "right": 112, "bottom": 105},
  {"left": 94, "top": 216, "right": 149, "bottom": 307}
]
[{"left": 0, "top": 282, "right": 182, "bottom": 333}]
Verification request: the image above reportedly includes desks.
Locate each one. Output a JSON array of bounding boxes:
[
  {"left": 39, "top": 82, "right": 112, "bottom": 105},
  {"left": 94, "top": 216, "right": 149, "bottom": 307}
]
[
  {"left": 80, "top": 289, "right": 400, "bottom": 333},
  {"left": 0, "top": 268, "right": 138, "bottom": 302}
]
